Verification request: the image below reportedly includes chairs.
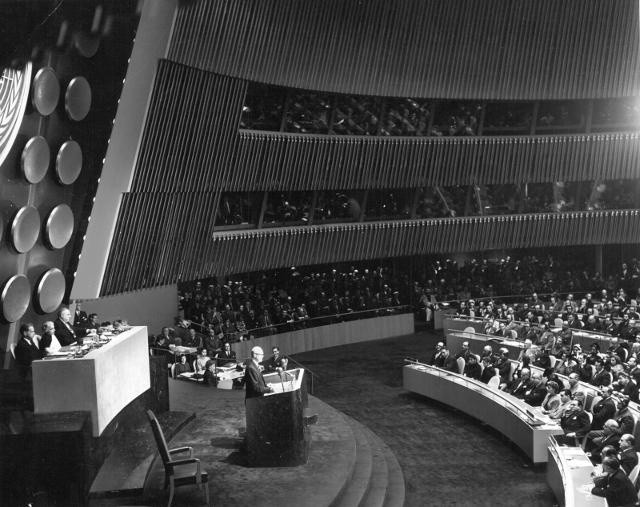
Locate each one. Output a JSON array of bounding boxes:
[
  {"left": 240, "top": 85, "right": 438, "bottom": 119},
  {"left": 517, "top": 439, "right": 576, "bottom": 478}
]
[
  {"left": 147, "top": 321, "right": 247, "bottom": 390},
  {"left": 8, "top": 334, "right": 44, "bottom": 409},
  {"left": 146, "top": 410, "right": 210, "bottom": 507},
  {"left": 403, "top": 289, "right": 638, "bottom": 507}
]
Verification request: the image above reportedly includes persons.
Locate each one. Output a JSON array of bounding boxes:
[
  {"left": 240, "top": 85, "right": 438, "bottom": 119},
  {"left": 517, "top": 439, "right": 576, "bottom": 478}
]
[
  {"left": 15, "top": 303, "right": 100, "bottom": 376},
  {"left": 150, "top": 99, "right": 640, "bottom": 507}
]
